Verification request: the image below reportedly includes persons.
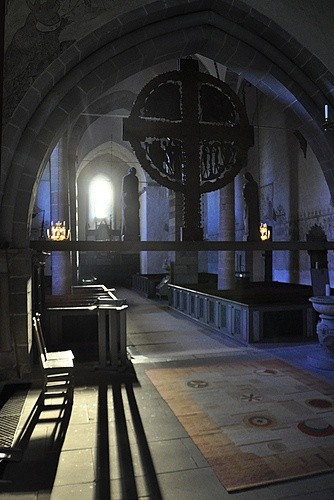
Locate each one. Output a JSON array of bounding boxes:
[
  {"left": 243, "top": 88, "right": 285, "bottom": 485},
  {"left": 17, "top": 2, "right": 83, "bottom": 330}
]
[
  {"left": 241, "top": 172, "right": 264, "bottom": 240},
  {"left": 119, "top": 167, "right": 149, "bottom": 242}
]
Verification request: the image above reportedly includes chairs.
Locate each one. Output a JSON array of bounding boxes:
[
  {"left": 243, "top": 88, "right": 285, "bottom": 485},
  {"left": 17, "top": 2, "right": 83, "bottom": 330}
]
[
  {"left": 36, "top": 312, "right": 75, "bottom": 360},
  {"left": 32, "top": 317, "right": 74, "bottom": 404}
]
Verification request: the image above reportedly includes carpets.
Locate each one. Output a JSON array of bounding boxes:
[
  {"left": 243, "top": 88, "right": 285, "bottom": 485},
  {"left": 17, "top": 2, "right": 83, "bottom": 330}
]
[{"left": 146, "top": 357, "right": 334, "bottom": 492}]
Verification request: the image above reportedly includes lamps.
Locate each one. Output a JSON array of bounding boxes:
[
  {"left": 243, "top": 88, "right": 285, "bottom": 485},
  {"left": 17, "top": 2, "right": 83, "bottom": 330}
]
[{"left": 47, "top": 221, "right": 71, "bottom": 240}]
